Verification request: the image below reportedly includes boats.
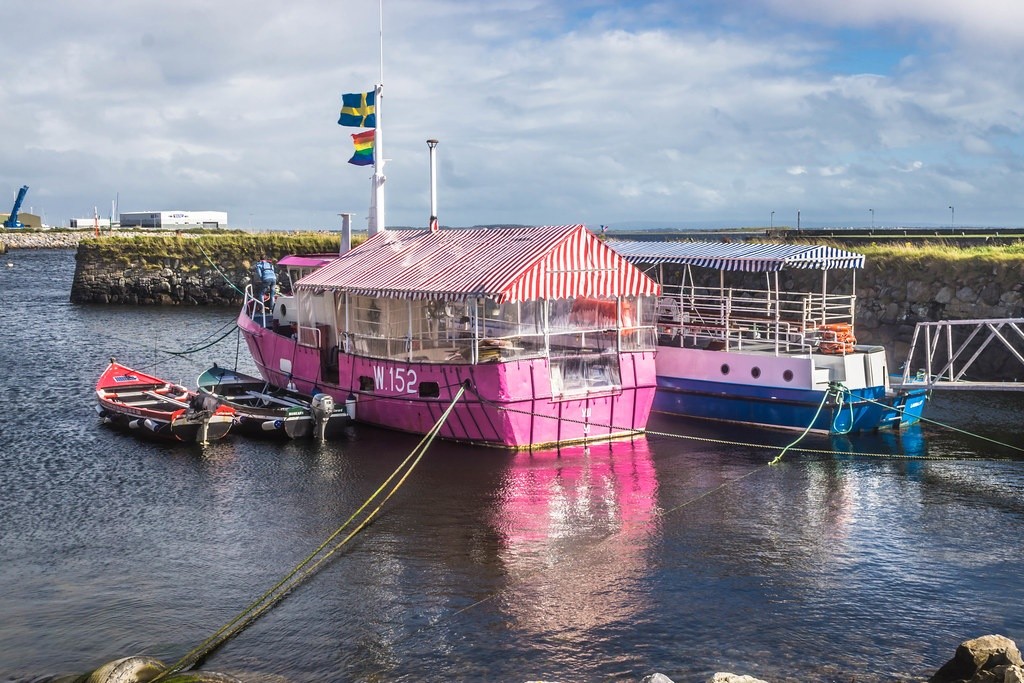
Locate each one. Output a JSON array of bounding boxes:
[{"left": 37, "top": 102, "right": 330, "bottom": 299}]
[
  {"left": 94, "top": 356, "right": 237, "bottom": 450},
  {"left": 235, "top": 1, "right": 665, "bottom": 452},
  {"left": 197, "top": 362, "right": 350, "bottom": 444},
  {"left": 598, "top": 236, "right": 927, "bottom": 436}
]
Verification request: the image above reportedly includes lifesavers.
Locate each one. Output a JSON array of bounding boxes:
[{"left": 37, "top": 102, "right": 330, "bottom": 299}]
[
  {"left": 818, "top": 322, "right": 856, "bottom": 354},
  {"left": 151, "top": 670, "right": 244, "bottom": 683},
  {"left": 84, "top": 655, "right": 168, "bottom": 683}
]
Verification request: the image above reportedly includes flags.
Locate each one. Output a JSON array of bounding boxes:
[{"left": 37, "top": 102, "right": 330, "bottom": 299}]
[
  {"left": 348, "top": 129, "right": 376, "bottom": 165},
  {"left": 339, "top": 91, "right": 376, "bottom": 128}
]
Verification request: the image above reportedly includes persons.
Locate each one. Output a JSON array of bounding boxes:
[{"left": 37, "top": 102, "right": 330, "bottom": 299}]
[{"left": 257, "top": 253, "right": 277, "bottom": 315}]
